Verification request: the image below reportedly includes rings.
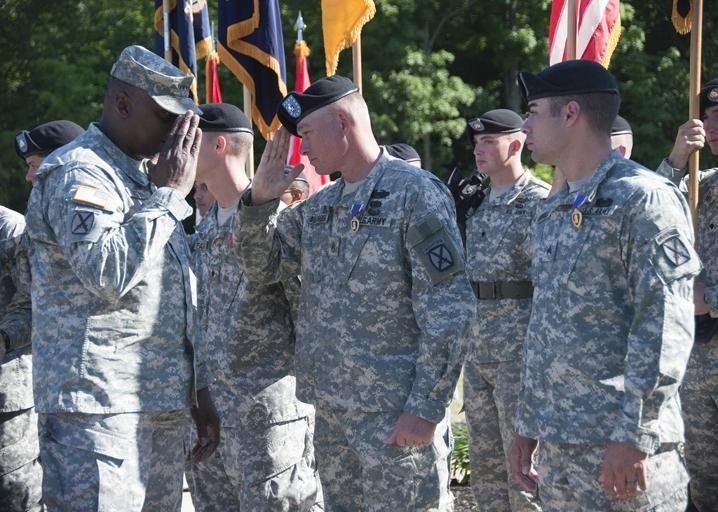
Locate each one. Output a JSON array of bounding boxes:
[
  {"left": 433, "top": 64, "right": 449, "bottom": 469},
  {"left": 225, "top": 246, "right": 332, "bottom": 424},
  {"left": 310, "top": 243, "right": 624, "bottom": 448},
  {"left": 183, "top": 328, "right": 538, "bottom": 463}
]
[{"left": 628, "top": 478, "right": 636, "bottom": 486}]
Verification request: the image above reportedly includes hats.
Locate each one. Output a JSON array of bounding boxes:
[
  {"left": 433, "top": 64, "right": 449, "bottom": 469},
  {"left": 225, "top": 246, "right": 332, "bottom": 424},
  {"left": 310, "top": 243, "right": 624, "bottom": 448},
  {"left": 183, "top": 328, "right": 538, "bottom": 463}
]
[
  {"left": 514, "top": 58, "right": 623, "bottom": 103},
  {"left": 13, "top": 118, "right": 89, "bottom": 160},
  {"left": 282, "top": 163, "right": 311, "bottom": 184},
  {"left": 381, "top": 141, "right": 423, "bottom": 164},
  {"left": 700, "top": 77, "right": 718, "bottom": 115},
  {"left": 608, "top": 112, "right": 633, "bottom": 136},
  {"left": 192, "top": 101, "right": 258, "bottom": 137},
  {"left": 466, "top": 108, "right": 529, "bottom": 136},
  {"left": 107, "top": 43, "right": 207, "bottom": 117},
  {"left": 274, "top": 71, "right": 361, "bottom": 140}
]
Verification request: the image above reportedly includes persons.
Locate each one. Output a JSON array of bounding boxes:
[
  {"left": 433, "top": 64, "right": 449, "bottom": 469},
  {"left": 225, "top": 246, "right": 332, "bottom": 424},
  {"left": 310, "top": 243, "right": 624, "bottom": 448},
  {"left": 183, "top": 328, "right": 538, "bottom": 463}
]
[
  {"left": 547, "top": 114, "right": 635, "bottom": 200},
  {"left": 510, "top": 61, "right": 703, "bottom": 512},
  {"left": 1, "top": 206, "right": 44, "bottom": 511},
  {"left": 188, "top": 104, "right": 321, "bottom": 510},
  {"left": 382, "top": 143, "right": 421, "bottom": 169},
  {"left": 463, "top": 108, "right": 555, "bottom": 512},
  {"left": 28, "top": 46, "right": 220, "bottom": 512},
  {"left": 1, "top": 120, "right": 87, "bottom": 362},
  {"left": 650, "top": 81, "right": 718, "bottom": 512},
  {"left": 230, "top": 75, "right": 477, "bottom": 512}
]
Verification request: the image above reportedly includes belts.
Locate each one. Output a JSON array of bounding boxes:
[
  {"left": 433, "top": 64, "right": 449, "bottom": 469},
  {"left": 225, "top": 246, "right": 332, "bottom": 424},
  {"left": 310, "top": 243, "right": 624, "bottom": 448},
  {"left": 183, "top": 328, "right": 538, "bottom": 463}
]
[{"left": 468, "top": 278, "right": 536, "bottom": 300}]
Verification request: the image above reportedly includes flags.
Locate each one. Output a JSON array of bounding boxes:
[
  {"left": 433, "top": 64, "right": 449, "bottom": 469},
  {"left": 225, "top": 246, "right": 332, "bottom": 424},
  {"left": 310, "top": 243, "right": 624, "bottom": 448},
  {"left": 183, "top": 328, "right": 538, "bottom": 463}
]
[
  {"left": 547, "top": 0, "right": 622, "bottom": 70},
  {"left": 146, "top": 1, "right": 377, "bottom": 208},
  {"left": 672, "top": 0, "right": 693, "bottom": 37}
]
